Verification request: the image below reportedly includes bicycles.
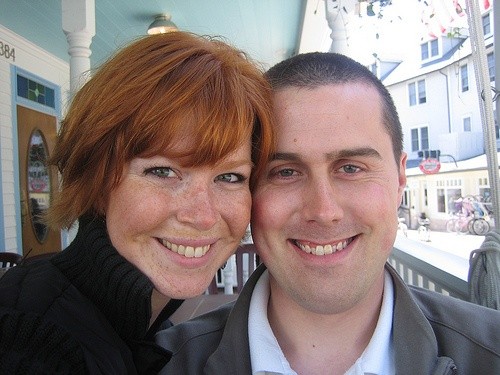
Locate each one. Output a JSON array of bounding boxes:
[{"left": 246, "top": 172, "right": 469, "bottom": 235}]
[{"left": 445, "top": 195, "right": 495, "bottom": 236}]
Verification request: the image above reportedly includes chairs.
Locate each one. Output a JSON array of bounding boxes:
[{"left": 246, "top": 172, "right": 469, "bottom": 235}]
[{"left": 0, "top": 251, "right": 24, "bottom": 280}]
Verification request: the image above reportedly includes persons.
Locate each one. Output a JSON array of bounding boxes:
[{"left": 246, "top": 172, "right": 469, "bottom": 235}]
[
  {"left": 155, "top": 51, "right": 500, "bottom": 375},
  {"left": 0, "top": 33, "right": 278, "bottom": 375}
]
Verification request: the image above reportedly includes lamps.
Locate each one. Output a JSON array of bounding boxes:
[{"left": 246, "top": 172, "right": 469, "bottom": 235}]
[{"left": 147, "top": 13, "right": 179, "bottom": 35}]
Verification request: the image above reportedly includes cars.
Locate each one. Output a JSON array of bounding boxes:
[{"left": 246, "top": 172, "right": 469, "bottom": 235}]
[{"left": 397, "top": 205, "right": 430, "bottom": 231}]
